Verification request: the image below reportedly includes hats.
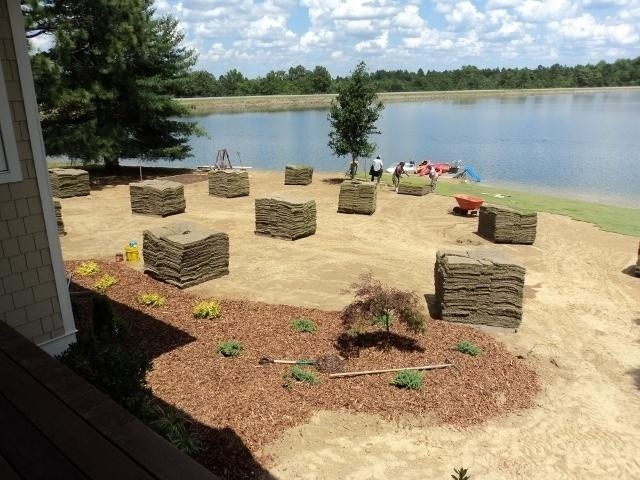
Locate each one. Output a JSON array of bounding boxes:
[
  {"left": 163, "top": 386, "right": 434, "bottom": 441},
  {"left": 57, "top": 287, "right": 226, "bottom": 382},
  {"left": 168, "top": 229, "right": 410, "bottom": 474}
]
[
  {"left": 377, "top": 155, "right": 380, "bottom": 158},
  {"left": 422, "top": 160, "right": 427, "bottom": 163}
]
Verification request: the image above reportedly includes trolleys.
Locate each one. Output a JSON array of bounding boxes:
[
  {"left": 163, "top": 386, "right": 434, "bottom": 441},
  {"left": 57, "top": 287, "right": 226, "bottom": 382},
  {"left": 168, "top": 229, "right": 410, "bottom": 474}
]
[{"left": 453, "top": 194, "right": 485, "bottom": 216}]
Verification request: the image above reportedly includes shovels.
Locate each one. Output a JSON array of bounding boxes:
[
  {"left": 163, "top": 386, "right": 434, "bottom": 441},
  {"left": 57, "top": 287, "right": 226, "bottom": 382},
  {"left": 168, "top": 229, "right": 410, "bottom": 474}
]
[{"left": 258, "top": 356, "right": 345, "bottom": 365}]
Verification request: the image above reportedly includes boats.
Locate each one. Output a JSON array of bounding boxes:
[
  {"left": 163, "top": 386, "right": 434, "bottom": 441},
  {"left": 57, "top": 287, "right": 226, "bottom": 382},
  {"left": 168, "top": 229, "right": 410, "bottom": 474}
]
[
  {"left": 386, "top": 161, "right": 451, "bottom": 174},
  {"left": 197, "top": 165, "right": 253, "bottom": 171}
]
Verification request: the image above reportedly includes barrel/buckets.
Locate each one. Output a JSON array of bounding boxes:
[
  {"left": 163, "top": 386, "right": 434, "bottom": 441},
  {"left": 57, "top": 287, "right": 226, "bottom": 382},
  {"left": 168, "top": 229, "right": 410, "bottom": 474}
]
[{"left": 125, "top": 246, "right": 140, "bottom": 262}]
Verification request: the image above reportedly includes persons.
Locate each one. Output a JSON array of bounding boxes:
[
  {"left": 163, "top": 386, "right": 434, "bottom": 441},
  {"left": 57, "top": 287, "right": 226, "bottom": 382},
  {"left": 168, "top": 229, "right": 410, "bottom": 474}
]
[
  {"left": 419, "top": 161, "right": 430, "bottom": 176},
  {"left": 427, "top": 165, "right": 439, "bottom": 193},
  {"left": 370, "top": 155, "right": 383, "bottom": 183},
  {"left": 392, "top": 161, "right": 409, "bottom": 193}
]
